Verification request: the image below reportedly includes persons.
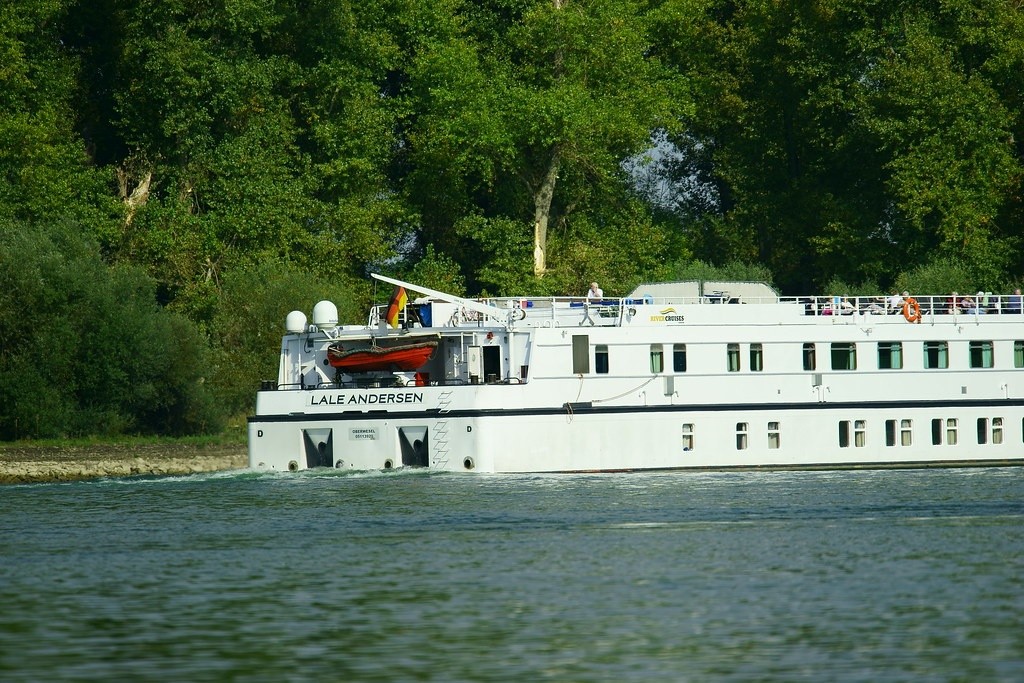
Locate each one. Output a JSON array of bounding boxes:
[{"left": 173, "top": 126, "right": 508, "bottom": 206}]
[
  {"left": 820, "top": 294, "right": 857, "bottom": 316},
  {"left": 944, "top": 289, "right": 1022, "bottom": 315},
  {"left": 588, "top": 281, "right": 604, "bottom": 303},
  {"left": 865, "top": 289, "right": 915, "bottom": 316}
]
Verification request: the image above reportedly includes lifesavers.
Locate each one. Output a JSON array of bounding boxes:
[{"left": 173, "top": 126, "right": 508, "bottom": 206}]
[{"left": 903, "top": 298, "right": 920, "bottom": 321}]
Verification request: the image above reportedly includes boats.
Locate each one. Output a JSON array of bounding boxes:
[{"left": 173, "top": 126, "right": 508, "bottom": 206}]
[{"left": 248, "top": 268, "right": 1023, "bottom": 480}]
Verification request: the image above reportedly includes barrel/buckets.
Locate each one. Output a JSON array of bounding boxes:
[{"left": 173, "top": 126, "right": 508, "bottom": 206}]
[
  {"left": 261, "top": 380, "right": 275, "bottom": 390},
  {"left": 402, "top": 323, "right": 407, "bottom": 329},
  {"left": 406, "top": 321, "right": 413, "bottom": 328},
  {"left": 308, "top": 385, "right": 316, "bottom": 390},
  {"left": 513, "top": 300, "right": 533, "bottom": 308},
  {"left": 488, "top": 374, "right": 496, "bottom": 384},
  {"left": 471, "top": 376, "right": 478, "bottom": 384}
]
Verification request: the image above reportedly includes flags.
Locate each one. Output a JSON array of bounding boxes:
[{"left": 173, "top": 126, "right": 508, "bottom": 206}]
[{"left": 386, "top": 274, "right": 409, "bottom": 331}]
